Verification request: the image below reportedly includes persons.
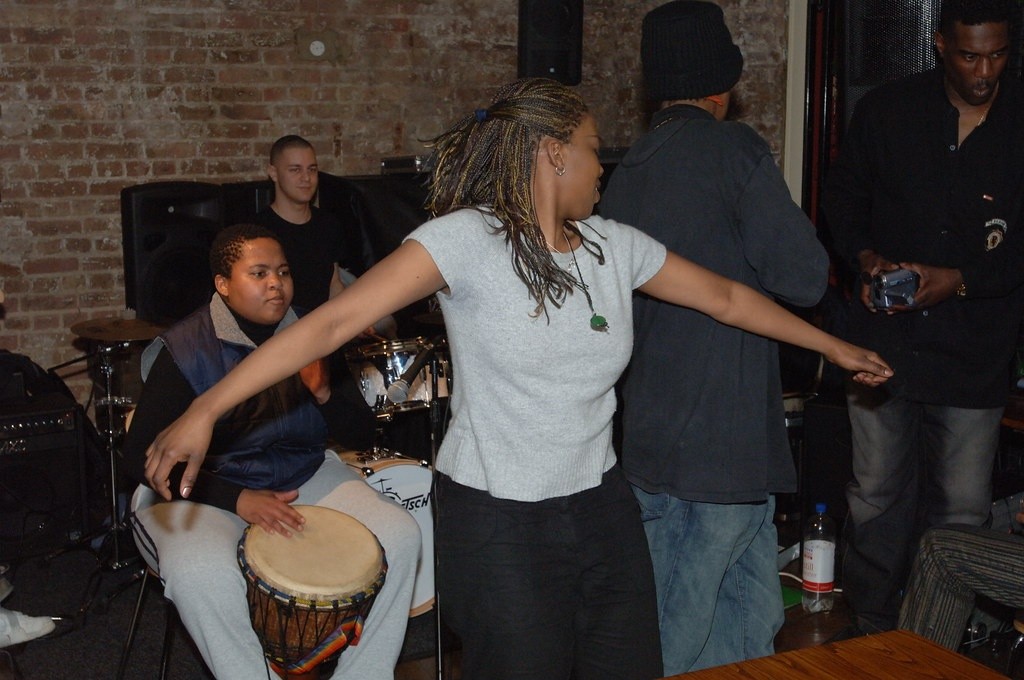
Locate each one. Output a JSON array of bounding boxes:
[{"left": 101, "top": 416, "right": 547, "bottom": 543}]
[
  {"left": 145, "top": 77, "right": 893, "bottom": 680},
  {"left": 824, "top": 0, "right": 1024, "bottom": 642},
  {"left": 127, "top": 221, "right": 423, "bottom": 680},
  {"left": 244, "top": 136, "right": 377, "bottom": 340},
  {"left": 896, "top": 512, "right": 1024, "bottom": 652},
  {"left": 598, "top": 0, "right": 831, "bottom": 678}
]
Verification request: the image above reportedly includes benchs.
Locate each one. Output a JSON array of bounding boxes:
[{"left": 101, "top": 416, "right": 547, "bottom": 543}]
[{"left": 119, "top": 160, "right": 620, "bottom": 348}]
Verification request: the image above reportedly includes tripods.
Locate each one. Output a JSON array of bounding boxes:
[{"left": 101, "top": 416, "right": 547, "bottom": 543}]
[{"left": 31, "top": 342, "right": 143, "bottom": 628}]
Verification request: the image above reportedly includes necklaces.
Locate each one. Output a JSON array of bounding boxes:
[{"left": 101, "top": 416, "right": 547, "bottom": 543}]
[
  {"left": 540, "top": 231, "right": 608, "bottom": 330},
  {"left": 958, "top": 102, "right": 992, "bottom": 146}
]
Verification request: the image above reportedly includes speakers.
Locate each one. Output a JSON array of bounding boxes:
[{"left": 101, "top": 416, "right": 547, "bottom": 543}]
[
  {"left": 0, "top": 394, "right": 89, "bottom": 565},
  {"left": 120, "top": 179, "right": 221, "bottom": 314},
  {"left": 517, "top": 1, "right": 583, "bottom": 86}
]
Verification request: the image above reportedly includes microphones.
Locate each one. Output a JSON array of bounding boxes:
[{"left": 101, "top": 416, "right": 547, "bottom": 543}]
[{"left": 387, "top": 335, "right": 440, "bottom": 404}]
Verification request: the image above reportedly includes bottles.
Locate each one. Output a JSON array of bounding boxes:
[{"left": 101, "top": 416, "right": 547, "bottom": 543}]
[{"left": 802, "top": 504, "right": 836, "bottom": 613}]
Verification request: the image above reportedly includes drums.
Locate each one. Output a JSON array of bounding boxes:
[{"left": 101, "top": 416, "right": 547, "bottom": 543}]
[
  {"left": 235, "top": 504, "right": 387, "bottom": 680},
  {"left": 349, "top": 335, "right": 452, "bottom": 422},
  {"left": 337, "top": 448, "right": 436, "bottom": 619}
]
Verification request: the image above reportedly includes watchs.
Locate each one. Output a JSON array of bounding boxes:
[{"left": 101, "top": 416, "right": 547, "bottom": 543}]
[{"left": 955, "top": 279, "right": 967, "bottom": 298}]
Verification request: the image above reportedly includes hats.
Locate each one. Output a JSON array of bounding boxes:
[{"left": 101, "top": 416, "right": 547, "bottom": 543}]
[{"left": 640, "top": 0, "right": 744, "bottom": 101}]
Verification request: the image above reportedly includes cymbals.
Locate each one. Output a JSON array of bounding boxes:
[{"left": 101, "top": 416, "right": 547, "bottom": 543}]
[{"left": 70, "top": 317, "right": 166, "bottom": 342}]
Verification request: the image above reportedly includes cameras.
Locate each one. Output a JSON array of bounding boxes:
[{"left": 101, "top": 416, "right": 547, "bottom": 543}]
[{"left": 871, "top": 266, "right": 919, "bottom": 309}]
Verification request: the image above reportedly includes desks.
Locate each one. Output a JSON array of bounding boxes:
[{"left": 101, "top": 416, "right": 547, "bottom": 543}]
[{"left": 660, "top": 630, "right": 1011, "bottom": 680}]
